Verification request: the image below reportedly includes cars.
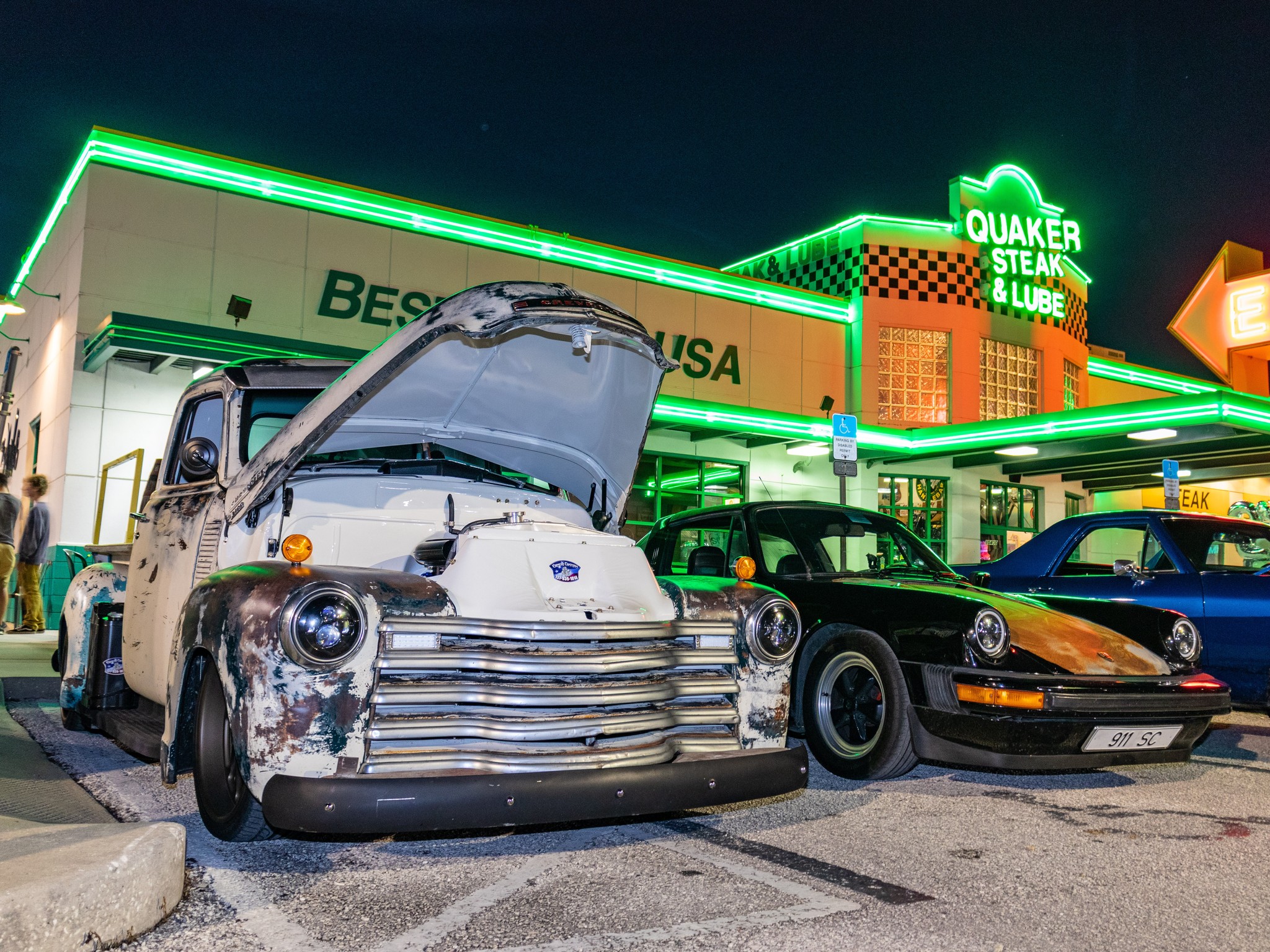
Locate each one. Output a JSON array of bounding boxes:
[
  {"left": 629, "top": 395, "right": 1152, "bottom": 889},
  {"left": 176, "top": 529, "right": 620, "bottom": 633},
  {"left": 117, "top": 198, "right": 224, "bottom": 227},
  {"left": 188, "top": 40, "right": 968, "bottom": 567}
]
[
  {"left": 633, "top": 497, "right": 1233, "bottom": 781},
  {"left": 880, "top": 509, "right": 1270, "bottom": 706},
  {"left": 50, "top": 277, "right": 808, "bottom": 843}
]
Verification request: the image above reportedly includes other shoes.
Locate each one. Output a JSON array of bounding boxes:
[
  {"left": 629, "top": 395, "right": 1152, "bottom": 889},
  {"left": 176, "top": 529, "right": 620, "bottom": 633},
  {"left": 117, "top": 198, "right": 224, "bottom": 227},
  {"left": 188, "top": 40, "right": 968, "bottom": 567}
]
[{"left": 0, "top": 621, "right": 7, "bottom": 635}]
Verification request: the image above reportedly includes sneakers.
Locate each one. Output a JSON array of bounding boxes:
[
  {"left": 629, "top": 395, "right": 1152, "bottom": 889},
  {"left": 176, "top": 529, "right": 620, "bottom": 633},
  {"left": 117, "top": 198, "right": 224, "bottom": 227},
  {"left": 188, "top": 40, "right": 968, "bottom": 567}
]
[
  {"left": 36, "top": 628, "right": 45, "bottom": 634},
  {"left": 5, "top": 624, "right": 37, "bottom": 634}
]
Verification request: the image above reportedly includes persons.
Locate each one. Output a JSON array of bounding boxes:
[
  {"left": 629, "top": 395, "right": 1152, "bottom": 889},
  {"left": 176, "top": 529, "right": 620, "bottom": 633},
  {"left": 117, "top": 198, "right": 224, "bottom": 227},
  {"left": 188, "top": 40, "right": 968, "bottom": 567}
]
[
  {"left": 0, "top": 473, "right": 24, "bottom": 634},
  {"left": 7, "top": 474, "right": 51, "bottom": 634}
]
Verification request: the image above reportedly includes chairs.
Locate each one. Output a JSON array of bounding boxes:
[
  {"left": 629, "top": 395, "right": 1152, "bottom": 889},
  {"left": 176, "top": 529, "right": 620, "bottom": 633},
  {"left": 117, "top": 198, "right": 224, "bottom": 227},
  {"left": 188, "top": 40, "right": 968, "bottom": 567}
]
[
  {"left": 60, "top": 546, "right": 89, "bottom": 585},
  {"left": 8, "top": 559, "right": 51, "bottom": 631}
]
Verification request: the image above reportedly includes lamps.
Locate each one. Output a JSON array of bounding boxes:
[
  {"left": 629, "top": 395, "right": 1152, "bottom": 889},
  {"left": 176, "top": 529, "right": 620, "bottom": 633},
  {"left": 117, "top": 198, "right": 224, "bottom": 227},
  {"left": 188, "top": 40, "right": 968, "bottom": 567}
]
[
  {"left": 0, "top": 281, "right": 60, "bottom": 317},
  {"left": 818, "top": 394, "right": 834, "bottom": 419},
  {"left": 227, "top": 293, "right": 251, "bottom": 328}
]
